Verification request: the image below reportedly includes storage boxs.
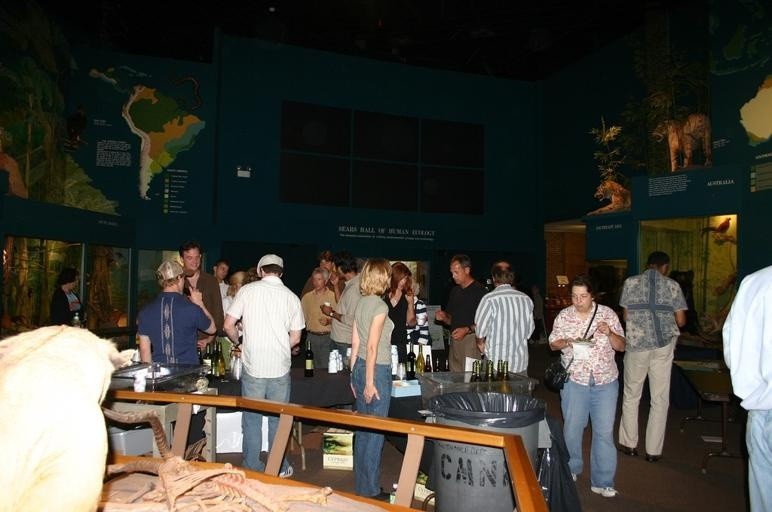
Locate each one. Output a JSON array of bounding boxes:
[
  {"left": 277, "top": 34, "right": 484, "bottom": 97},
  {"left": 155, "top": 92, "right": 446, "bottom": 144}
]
[
  {"left": 110, "top": 362, "right": 208, "bottom": 405},
  {"left": 415, "top": 371, "right": 540, "bottom": 412}
]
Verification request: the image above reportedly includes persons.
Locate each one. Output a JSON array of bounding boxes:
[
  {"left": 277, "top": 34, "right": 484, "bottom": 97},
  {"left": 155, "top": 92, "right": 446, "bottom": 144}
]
[
  {"left": 531, "top": 285, "right": 543, "bottom": 344},
  {"left": 349, "top": 257, "right": 396, "bottom": 501},
  {"left": 222, "top": 253, "right": 306, "bottom": 481},
  {"left": 179, "top": 240, "right": 225, "bottom": 352},
  {"left": 138, "top": 259, "right": 217, "bottom": 448},
  {"left": 548, "top": 273, "right": 627, "bottom": 497},
  {"left": 474, "top": 261, "right": 537, "bottom": 373},
  {"left": 50, "top": 266, "right": 89, "bottom": 328},
  {"left": 434, "top": 255, "right": 491, "bottom": 371},
  {"left": 720, "top": 264, "right": 770, "bottom": 512},
  {"left": 616, "top": 251, "right": 689, "bottom": 461},
  {"left": 200, "top": 251, "right": 432, "bottom": 377}
]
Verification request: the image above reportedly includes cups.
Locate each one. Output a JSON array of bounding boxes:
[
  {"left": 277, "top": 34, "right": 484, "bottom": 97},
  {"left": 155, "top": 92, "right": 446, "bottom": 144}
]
[
  {"left": 133, "top": 374, "right": 147, "bottom": 393},
  {"left": 324, "top": 302, "right": 331, "bottom": 307}
]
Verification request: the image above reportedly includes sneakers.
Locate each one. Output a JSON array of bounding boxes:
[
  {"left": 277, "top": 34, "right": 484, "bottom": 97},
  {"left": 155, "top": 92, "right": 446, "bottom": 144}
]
[
  {"left": 279, "top": 466, "right": 293, "bottom": 478},
  {"left": 376, "top": 492, "right": 389, "bottom": 500},
  {"left": 591, "top": 486, "right": 618, "bottom": 497}
]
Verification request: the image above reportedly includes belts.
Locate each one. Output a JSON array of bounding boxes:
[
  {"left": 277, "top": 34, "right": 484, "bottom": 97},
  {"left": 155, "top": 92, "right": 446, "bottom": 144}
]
[{"left": 308, "top": 331, "right": 329, "bottom": 335}]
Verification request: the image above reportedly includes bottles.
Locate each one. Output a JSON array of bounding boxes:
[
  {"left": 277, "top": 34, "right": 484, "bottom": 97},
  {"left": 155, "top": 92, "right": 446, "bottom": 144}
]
[
  {"left": 303, "top": 339, "right": 314, "bottom": 378},
  {"left": 197, "top": 340, "right": 242, "bottom": 381},
  {"left": 81, "top": 313, "right": 89, "bottom": 328},
  {"left": 470, "top": 360, "right": 512, "bottom": 394},
  {"left": 73, "top": 313, "right": 80, "bottom": 327},
  {"left": 327, "top": 347, "right": 351, "bottom": 374},
  {"left": 390, "top": 342, "right": 449, "bottom": 380}
]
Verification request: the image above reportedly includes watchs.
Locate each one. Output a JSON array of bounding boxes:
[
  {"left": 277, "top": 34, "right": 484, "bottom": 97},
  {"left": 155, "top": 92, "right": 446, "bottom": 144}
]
[{"left": 466, "top": 324, "right": 473, "bottom": 335}]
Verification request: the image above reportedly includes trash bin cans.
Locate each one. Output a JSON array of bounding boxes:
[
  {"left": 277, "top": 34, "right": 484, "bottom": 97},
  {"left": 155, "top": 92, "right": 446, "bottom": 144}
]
[{"left": 427, "top": 392, "right": 545, "bottom": 512}]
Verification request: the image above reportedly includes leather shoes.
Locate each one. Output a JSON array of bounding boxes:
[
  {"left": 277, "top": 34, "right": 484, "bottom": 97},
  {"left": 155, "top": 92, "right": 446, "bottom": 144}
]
[
  {"left": 646, "top": 454, "right": 656, "bottom": 461},
  {"left": 615, "top": 440, "right": 635, "bottom": 456}
]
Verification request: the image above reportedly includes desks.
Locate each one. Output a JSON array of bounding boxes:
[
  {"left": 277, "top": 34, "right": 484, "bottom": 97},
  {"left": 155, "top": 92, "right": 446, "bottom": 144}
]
[
  {"left": 388, "top": 395, "right": 434, "bottom": 474},
  {"left": 673, "top": 360, "right": 748, "bottom": 458},
  {"left": 206, "top": 367, "right": 355, "bottom": 472}
]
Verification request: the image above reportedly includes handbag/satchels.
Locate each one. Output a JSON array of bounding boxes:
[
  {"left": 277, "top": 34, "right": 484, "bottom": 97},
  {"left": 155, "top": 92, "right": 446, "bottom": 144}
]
[{"left": 544, "top": 361, "right": 567, "bottom": 392}]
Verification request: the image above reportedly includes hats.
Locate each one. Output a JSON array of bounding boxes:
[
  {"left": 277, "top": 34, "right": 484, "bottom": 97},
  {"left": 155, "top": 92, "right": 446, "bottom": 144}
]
[
  {"left": 257, "top": 254, "right": 284, "bottom": 277},
  {"left": 155, "top": 260, "right": 194, "bottom": 281}
]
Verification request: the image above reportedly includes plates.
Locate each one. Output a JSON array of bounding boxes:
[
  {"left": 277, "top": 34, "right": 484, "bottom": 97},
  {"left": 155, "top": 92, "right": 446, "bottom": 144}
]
[{"left": 569, "top": 342, "right": 597, "bottom": 349}]
